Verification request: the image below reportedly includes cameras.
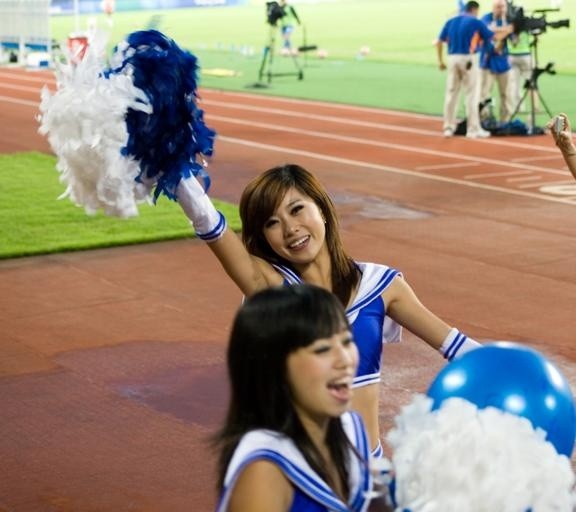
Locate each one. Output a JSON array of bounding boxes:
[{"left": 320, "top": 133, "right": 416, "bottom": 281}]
[{"left": 553, "top": 116, "right": 566, "bottom": 132}]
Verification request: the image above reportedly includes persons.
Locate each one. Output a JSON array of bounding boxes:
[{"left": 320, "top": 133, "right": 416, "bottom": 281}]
[
  {"left": 505, "top": 30, "right": 548, "bottom": 114},
  {"left": 545, "top": 112, "right": 576, "bottom": 180},
  {"left": 159, "top": 162, "right": 483, "bottom": 512},
  {"left": 475, "top": 0, "right": 521, "bottom": 126},
  {"left": 203, "top": 282, "right": 391, "bottom": 510},
  {"left": 432, "top": 3, "right": 516, "bottom": 140}
]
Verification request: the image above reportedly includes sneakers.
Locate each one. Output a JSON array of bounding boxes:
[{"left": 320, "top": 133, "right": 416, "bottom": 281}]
[
  {"left": 467, "top": 128, "right": 491, "bottom": 138},
  {"left": 445, "top": 128, "right": 453, "bottom": 137}
]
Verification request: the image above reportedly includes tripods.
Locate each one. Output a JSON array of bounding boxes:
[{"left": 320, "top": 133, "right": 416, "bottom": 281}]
[
  {"left": 259, "top": 27, "right": 303, "bottom": 84},
  {"left": 508, "top": 37, "right": 555, "bottom": 135}
]
